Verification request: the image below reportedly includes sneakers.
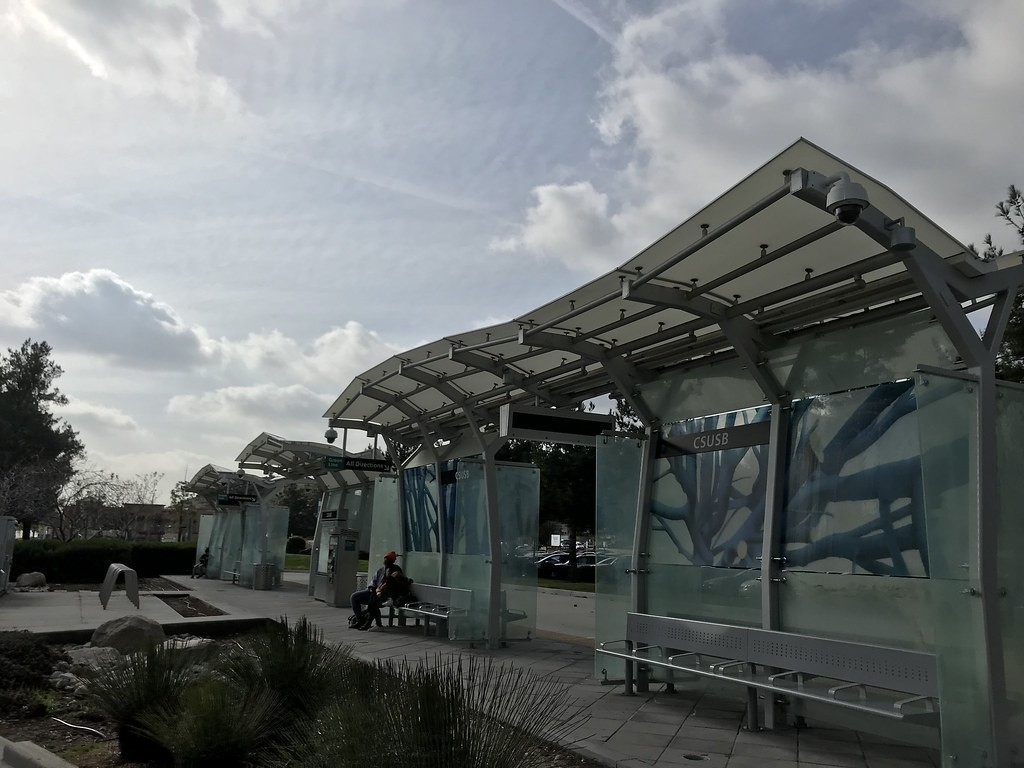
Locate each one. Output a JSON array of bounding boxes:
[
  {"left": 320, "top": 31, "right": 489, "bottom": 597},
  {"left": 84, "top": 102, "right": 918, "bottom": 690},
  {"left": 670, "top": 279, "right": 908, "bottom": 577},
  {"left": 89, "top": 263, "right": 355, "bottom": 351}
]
[{"left": 367, "top": 624, "right": 384, "bottom": 632}]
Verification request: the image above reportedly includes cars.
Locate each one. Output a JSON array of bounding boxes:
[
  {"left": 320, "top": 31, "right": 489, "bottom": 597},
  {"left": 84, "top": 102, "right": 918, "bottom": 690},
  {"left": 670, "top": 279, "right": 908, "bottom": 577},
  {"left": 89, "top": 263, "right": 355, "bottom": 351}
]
[{"left": 510, "top": 539, "right": 636, "bottom": 584}]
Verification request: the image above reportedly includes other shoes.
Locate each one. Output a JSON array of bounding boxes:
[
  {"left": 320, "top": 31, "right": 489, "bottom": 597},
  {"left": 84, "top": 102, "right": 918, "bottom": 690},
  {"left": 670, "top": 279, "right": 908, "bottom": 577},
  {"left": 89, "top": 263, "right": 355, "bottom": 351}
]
[
  {"left": 197, "top": 575, "right": 200, "bottom": 579},
  {"left": 191, "top": 575, "right": 194, "bottom": 578}
]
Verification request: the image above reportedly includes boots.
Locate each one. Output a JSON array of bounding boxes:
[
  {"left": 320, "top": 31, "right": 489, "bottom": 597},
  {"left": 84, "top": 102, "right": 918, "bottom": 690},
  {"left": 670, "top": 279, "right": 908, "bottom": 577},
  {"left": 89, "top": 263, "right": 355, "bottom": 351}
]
[
  {"left": 348, "top": 605, "right": 362, "bottom": 629},
  {"left": 359, "top": 612, "right": 374, "bottom": 630}
]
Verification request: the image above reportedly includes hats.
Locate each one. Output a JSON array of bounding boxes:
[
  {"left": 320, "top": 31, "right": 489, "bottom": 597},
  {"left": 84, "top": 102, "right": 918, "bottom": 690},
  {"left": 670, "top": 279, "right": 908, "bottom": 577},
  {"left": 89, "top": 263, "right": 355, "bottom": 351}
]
[{"left": 384, "top": 552, "right": 396, "bottom": 559}]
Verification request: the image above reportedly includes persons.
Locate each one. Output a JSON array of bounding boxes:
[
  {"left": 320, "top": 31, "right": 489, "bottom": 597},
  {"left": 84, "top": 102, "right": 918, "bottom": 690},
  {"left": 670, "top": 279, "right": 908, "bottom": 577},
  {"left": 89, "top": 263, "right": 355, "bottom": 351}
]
[
  {"left": 190, "top": 548, "right": 213, "bottom": 580},
  {"left": 367, "top": 564, "right": 414, "bottom": 632},
  {"left": 348, "top": 551, "right": 403, "bottom": 632}
]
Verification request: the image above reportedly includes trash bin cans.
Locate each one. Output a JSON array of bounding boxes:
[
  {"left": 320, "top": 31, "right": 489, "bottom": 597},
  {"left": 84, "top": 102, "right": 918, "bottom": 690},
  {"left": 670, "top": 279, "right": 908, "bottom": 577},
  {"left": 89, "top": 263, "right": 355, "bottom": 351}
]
[{"left": 252, "top": 562, "right": 275, "bottom": 590}]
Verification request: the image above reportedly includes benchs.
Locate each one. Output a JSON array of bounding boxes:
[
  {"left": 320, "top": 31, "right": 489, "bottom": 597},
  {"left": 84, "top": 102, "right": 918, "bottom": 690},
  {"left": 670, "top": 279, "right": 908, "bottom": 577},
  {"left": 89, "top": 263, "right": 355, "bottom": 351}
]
[
  {"left": 595, "top": 610, "right": 940, "bottom": 743},
  {"left": 387, "top": 582, "right": 474, "bottom": 642},
  {"left": 223, "top": 560, "right": 241, "bottom": 585}
]
